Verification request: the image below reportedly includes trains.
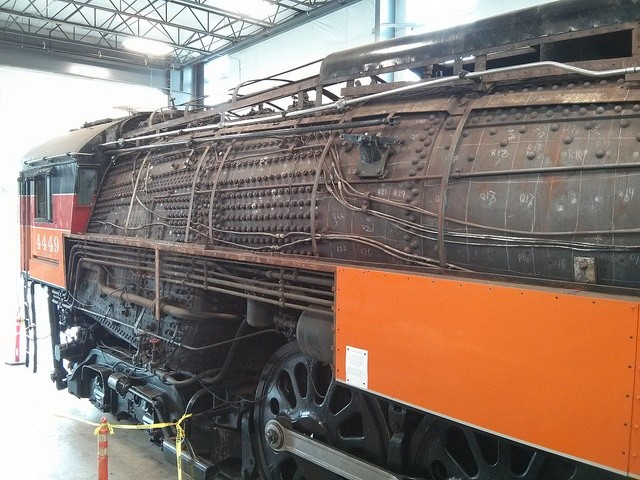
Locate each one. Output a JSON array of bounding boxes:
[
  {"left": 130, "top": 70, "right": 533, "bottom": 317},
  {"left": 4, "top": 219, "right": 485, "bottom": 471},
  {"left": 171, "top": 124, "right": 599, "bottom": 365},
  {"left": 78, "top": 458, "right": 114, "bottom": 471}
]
[{"left": 17, "top": 1, "right": 639, "bottom": 480}]
